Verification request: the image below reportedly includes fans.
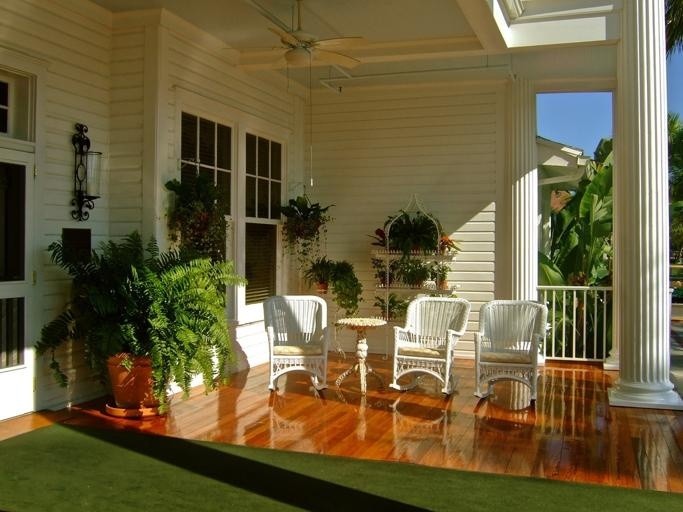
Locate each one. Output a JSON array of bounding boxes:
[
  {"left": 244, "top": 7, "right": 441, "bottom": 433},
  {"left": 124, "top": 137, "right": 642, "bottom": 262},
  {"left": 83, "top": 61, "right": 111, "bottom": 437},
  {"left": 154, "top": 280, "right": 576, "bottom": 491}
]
[{"left": 232, "top": 0, "right": 368, "bottom": 75}]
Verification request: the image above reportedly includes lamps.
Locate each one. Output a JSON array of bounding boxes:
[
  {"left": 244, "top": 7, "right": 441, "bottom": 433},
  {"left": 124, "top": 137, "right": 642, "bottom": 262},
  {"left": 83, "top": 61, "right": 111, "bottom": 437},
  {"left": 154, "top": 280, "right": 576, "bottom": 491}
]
[{"left": 71, "top": 122, "right": 102, "bottom": 221}]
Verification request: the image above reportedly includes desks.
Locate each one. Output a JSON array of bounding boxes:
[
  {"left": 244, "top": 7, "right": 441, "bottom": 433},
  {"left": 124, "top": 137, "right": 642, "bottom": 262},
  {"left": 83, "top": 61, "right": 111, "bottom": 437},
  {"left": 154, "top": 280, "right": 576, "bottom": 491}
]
[{"left": 334, "top": 318, "right": 388, "bottom": 396}]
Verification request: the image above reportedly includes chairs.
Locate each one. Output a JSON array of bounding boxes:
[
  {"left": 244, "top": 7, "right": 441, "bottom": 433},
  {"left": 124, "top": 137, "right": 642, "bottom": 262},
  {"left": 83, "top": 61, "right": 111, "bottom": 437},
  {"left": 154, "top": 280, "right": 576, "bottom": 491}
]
[
  {"left": 472, "top": 300, "right": 547, "bottom": 401},
  {"left": 388, "top": 389, "right": 454, "bottom": 464},
  {"left": 263, "top": 295, "right": 329, "bottom": 391},
  {"left": 268, "top": 393, "right": 326, "bottom": 454},
  {"left": 389, "top": 296, "right": 472, "bottom": 396},
  {"left": 470, "top": 398, "right": 537, "bottom": 469}
]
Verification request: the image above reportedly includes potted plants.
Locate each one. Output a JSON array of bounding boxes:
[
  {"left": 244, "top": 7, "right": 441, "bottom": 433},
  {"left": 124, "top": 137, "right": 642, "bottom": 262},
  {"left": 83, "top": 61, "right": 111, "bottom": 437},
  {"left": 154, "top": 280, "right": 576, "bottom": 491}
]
[
  {"left": 165, "top": 177, "right": 231, "bottom": 262},
  {"left": 373, "top": 292, "right": 400, "bottom": 320},
  {"left": 34, "top": 229, "right": 249, "bottom": 416},
  {"left": 429, "top": 262, "right": 452, "bottom": 290},
  {"left": 366, "top": 209, "right": 463, "bottom": 252},
  {"left": 396, "top": 257, "right": 430, "bottom": 288},
  {"left": 370, "top": 257, "right": 399, "bottom": 287},
  {"left": 302, "top": 255, "right": 367, "bottom": 317},
  {"left": 280, "top": 189, "right": 337, "bottom": 271}
]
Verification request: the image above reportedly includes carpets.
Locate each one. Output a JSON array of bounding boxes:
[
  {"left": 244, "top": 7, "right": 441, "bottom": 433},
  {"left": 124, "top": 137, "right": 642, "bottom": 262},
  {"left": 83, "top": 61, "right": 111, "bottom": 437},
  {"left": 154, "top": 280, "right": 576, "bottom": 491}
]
[{"left": 0, "top": 420, "right": 682, "bottom": 511}]
